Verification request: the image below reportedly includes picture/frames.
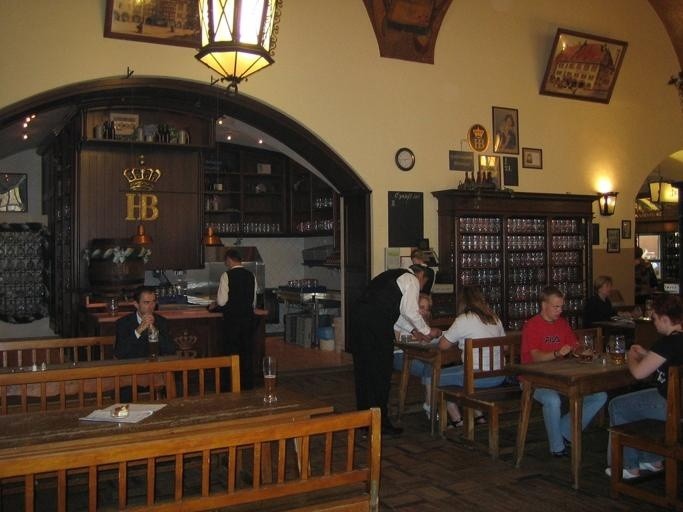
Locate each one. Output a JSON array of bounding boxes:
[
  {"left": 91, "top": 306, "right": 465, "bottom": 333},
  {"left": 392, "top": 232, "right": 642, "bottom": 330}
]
[
  {"left": 102, "top": 0, "right": 201, "bottom": 48},
  {"left": 521, "top": 147, "right": 542, "bottom": 168},
  {"left": 606, "top": 228, "right": 620, "bottom": 253},
  {"left": 491, "top": 105, "right": 518, "bottom": 155},
  {"left": 621, "top": 220, "right": 630, "bottom": 238},
  {"left": 539, "top": 27, "right": 627, "bottom": 105},
  {"left": 477, "top": 153, "right": 502, "bottom": 190}
]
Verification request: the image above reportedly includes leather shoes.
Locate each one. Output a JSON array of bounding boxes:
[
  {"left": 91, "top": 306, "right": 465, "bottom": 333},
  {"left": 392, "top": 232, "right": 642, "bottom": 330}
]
[{"left": 381, "top": 425, "right": 403, "bottom": 435}]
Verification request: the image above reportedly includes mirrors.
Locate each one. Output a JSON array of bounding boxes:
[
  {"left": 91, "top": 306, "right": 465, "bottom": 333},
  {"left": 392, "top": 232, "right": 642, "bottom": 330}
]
[{"left": 0, "top": 171, "right": 30, "bottom": 215}]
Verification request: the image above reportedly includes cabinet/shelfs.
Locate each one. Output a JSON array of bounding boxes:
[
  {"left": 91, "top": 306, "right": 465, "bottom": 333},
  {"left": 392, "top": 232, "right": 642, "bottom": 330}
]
[
  {"left": 431, "top": 182, "right": 596, "bottom": 335},
  {"left": 200, "top": 141, "right": 287, "bottom": 238},
  {"left": 33, "top": 121, "right": 73, "bottom": 337},
  {"left": 287, "top": 156, "right": 338, "bottom": 238}
]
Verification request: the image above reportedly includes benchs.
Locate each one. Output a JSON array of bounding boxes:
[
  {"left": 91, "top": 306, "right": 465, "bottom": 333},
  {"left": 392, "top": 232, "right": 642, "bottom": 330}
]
[
  {"left": 1, "top": 354, "right": 242, "bottom": 412},
  {"left": 436, "top": 328, "right": 603, "bottom": 460},
  {"left": 2, "top": 337, "right": 117, "bottom": 369},
  {"left": 1, "top": 405, "right": 382, "bottom": 512},
  {"left": 607, "top": 367, "right": 679, "bottom": 509}
]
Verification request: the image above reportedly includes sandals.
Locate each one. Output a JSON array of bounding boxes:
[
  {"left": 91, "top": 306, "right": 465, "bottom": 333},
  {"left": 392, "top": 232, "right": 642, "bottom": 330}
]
[
  {"left": 473, "top": 416, "right": 488, "bottom": 425},
  {"left": 443, "top": 416, "right": 465, "bottom": 429}
]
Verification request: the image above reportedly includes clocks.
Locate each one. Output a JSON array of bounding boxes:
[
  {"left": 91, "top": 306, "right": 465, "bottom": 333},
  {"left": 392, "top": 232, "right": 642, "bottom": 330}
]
[{"left": 394, "top": 147, "right": 414, "bottom": 170}]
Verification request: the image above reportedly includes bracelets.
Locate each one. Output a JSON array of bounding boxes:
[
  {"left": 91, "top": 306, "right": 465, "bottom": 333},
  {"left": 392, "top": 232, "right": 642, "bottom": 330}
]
[{"left": 554, "top": 350, "right": 559, "bottom": 358}]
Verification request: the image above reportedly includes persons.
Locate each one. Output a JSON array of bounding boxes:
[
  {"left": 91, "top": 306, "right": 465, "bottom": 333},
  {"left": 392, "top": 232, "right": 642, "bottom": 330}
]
[
  {"left": 436, "top": 283, "right": 510, "bottom": 430},
  {"left": 633, "top": 247, "right": 658, "bottom": 310},
  {"left": 520, "top": 285, "right": 608, "bottom": 459},
  {"left": 351, "top": 263, "right": 441, "bottom": 440},
  {"left": 497, "top": 115, "right": 517, "bottom": 152},
  {"left": 585, "top": 277, "right": 618, "bottom": 332},
  {"left": 391, "top": 292, "right": 451, "bottom": 425},
  {"left": 114, "top": 286, "right": 178, "bottom": 399},
  {"left": 604, "top": 292, "right": 683, "bottom": 481},
  {"left": 209, "top": 249, "right": 258, "bottom": 382}
]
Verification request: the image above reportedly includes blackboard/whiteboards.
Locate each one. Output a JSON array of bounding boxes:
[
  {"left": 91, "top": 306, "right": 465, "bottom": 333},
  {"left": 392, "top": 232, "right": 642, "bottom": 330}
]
[{"left": 388, "top": 191, "right": 423, "bottom": 247}]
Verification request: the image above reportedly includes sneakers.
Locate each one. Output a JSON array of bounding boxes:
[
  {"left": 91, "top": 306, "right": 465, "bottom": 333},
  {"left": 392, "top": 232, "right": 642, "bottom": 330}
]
[
  {"left": 423, "top": 402, "right": 439, "bottom": 421},
  {"left": 605, "top": 466, "right": 640, "bottom": 481},
  {"left": 551, "top": 434, "right": 574, "bottom": 457},
  {"left": 639, "top": 461, "right": 664, "bottom": 471}
]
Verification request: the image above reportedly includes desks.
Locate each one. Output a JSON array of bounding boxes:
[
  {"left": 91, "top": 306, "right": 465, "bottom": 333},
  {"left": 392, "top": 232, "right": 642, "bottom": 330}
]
[
  {"left": 155, "top": 303, "right": 269, "bottom": 384},
  {"left": 2, "top": 389, "right": 334, "bottom": 482},
  {"left": 1, "top": 355, "right": 176, "bottom": 397},
  {"left": 393, "top": 338, "right": 461, "bottom": 436},
  {"left": 505, "top": 351, "right": 655, "bottom": 490}
]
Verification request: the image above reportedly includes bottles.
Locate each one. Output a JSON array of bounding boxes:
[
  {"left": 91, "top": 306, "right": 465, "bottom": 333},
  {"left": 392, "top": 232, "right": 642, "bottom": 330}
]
[
  {"left": 147, "top": 324, "right": 160, "bottom": 361},
  {"left": 463, "top": 171, "right": 492, "bottom": 184}
]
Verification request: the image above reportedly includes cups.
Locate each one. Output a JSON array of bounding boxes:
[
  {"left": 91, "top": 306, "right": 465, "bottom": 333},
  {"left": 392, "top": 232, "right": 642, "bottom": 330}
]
[
  {"left": 573, "top": 334, "right": 593, "bottom": 364},
  {"left": 605, "top": 334, "right": 625, "bottom": 364},
  {"left": 106, "top": 296, "right": 118, "bottom": 317},
  {"left": 296, "top": 219, "right": 333, "bottom": 233},
  {"left": 459, "top": 218, "right": 583, "bottom": 329},
  {"left": 261, "top": 355, "right": 278, "bottom": 404},
  {"left": 315, "top": 197, "right": 333, "bottom": 209},
  {"left": 213, "top": 183, "right": 222, "bottom": 191},
  {"left": 205, "top": 222, "right": 279, "bottom": 232}
]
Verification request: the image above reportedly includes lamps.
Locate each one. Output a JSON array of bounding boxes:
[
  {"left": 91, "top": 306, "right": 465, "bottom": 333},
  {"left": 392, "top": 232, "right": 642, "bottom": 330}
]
[
  {"left": 195, "top": 178, "right": 223, "bottom": 246},
  {"left": 128, "top": 153, "right": 153, "bottom": 246},
  {"left": 595, "top": 189, "right": 618, "bottom": 216},
  {"left": 639, "top": 157, "right": 680, "bottom": 211},
  {"left": 192, "top": 0, "right": 287, "bottom": 99}
]
